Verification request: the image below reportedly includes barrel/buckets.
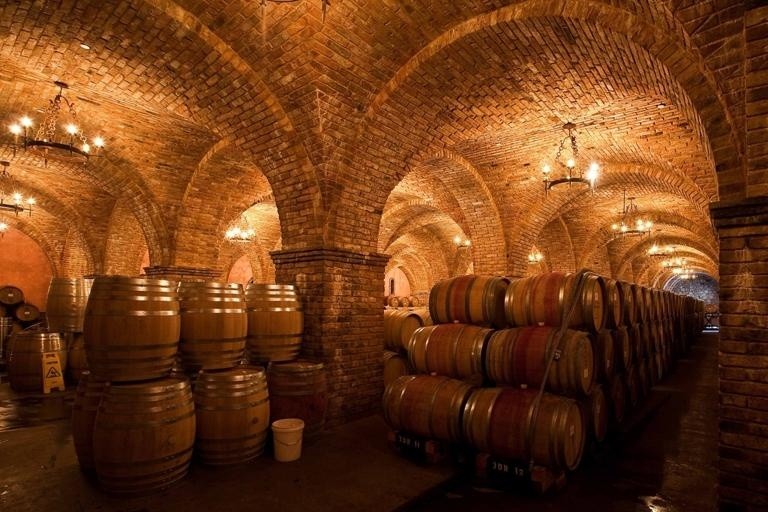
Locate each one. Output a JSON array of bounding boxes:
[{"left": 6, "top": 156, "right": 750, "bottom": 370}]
[
  {"left": 503, "top": 268, "right": 605, "bottom": 330},
  {"left": 383, "top": 349, "right": 410, "bottom": 389},
  {"left": 84, "top": 278, "right": 183, "bottom": 385},
  {"left": 409, "top": 324, "right": 483, "bottom": 379},
  {"left": 94, "top": 381, "right": 198, "bottom": 488},
  {"left": 381, "top": 310, "right": 421, "bottom": 352},
  {"left": 194, "top": 364, "right": 270, "bottom": 469},
  {"left": 271, "top": 417, "right": 305, "bottom": 461},
  {"left": 429, "top": 274, "right": 511, "bottom": 328},
  {"left": 12, "top": 304, "right": 40, "bottom": 329},
  {"left": 181, "top": 274, "right": 249, "bottom": 373},
  {"left": 9, "top": 333, "right": 63, "bottom": 390},
  {"left": 0, "top": 286, "right": 23, "bottom": 314},
  {"left": 407, "top": 296, "right": 419, "bottom": 306},
  {"left": 72, "top": 372, "right": 105, "bottom": 473},
  {"left": 46, "top": 276, "right": 92, "bottom": 330},
  {"left": 379, "top": 374, "right": 472, "bottom": 440},
  {"left": 484, "top": 326, "right": 593, "bottom": 398},
  {"left": 399, "top": 297, "right": 409, "bottom": 307},
  {"left": 462, "top": 385, "right": 586, "bottom": 473},
  {"left": 406, "top": 308, "right": 432, "bottom": 326},
  {"left": 68, "top": 333, "right": 89, "bottom": 374},
  {"left": 1, "top": 316, "right": 13, "bottom": 359},
  {"left": 244, "top": 282, "right": 303, "bottom": 362},
  {"left": 591, "top": 267, "right": 706, "bottom": 450},
  {"left": 385, "top": 296, "right": 399, "bottom": 307},
  {"left": 265, "top": 362, "right": 326, "bottom": 438}
]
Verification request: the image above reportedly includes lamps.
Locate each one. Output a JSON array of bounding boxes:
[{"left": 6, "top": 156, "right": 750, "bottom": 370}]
[
  {"left": 611, "top": 190, "right": 700, "bottom": 283},
  {"left": 454, "top": 231, "right": 470, "bottom": 249},
  {"left": 527, "top": 248, "right": 543, "bottom": 265},
  {"left": 542, "top": 123, "right": 601, "bottom": 198},
  {"left": 225, "top": 215, "right": 256, "bottom": 244},
  {"left": 0, "top": 81, "right": 104, "bottom": 234}
]
